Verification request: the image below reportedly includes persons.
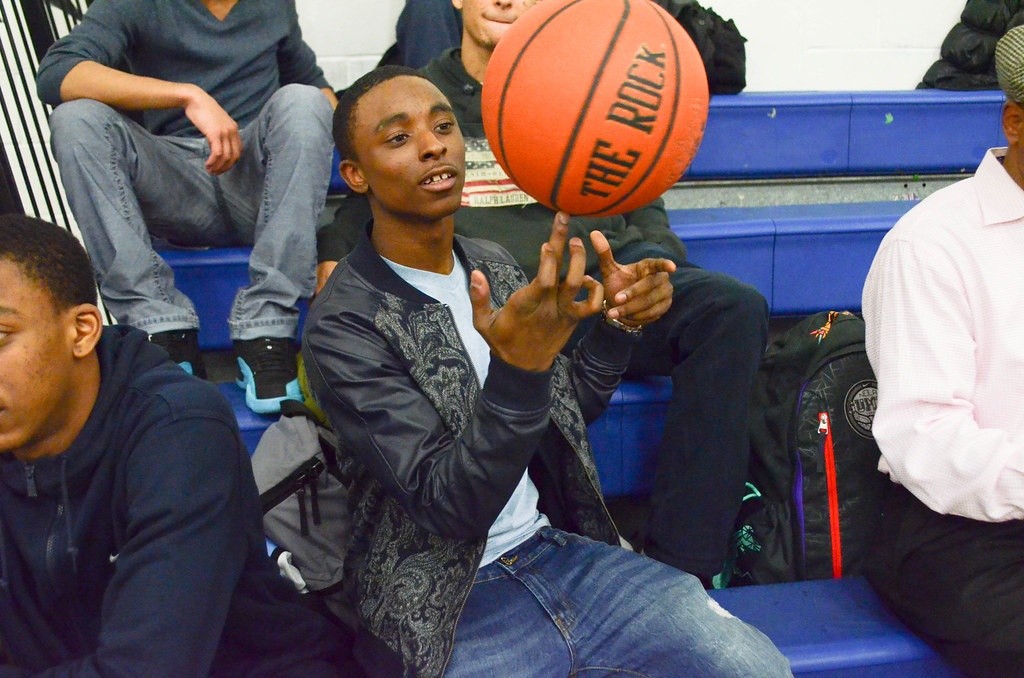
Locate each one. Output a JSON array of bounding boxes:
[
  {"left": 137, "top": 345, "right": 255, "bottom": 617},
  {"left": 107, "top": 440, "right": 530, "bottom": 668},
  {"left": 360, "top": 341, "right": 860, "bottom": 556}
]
[
  {"left": 316, "top": 0, "right": 769, "bottom": 593},
  {"left": 854, "top": 24, "right": 1024, "bottom": 678},
  {"left": 0, "top": 214, "right": 355, "bottom": 676},
  {"left": 302, "top": 65, "right": 795, "bottom": 678},
  {"left": 36, "top": 1, "right": 341, "bottom": 413}
]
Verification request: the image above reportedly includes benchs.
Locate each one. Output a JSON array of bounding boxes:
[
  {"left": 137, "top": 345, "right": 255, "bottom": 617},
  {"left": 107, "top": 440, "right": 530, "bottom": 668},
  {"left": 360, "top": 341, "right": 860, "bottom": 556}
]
[
  {"left": 159, "top": 203, "right": 922, "bottom": 356},
  {"left": 328, "top": 89, "right": 1007, "bottom": 196},
  {"left": 706, "top": 575, "right": 960, "bottom": 678},
  {"left": 215, "top": 374, "right": 678, "bottom": 497}
]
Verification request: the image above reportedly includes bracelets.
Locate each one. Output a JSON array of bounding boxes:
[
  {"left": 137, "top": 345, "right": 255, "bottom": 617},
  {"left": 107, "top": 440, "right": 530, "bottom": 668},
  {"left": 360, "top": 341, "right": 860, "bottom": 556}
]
[{"left": 602, "top": 298, "right": 642, "bottom": 335}]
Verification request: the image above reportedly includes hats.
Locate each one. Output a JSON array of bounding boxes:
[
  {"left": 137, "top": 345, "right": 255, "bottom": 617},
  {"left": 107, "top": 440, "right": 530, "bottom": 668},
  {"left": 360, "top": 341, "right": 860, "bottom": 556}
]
[{"left": 994, "top": 24, "right": 1024, "bottom": 104}]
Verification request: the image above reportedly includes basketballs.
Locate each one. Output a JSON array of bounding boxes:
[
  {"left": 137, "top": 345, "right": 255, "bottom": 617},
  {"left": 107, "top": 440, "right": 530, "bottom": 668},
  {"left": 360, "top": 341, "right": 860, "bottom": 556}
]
[{"left": 480, "top": 0, "right": 711, "bottom": 218}]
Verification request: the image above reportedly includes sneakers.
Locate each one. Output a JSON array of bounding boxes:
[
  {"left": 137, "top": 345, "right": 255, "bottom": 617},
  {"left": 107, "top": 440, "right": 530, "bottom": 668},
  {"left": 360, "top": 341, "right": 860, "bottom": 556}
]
[
  {"left": 147, "top": 329, "right": 208, "bottom": 381},
  {"left": 235, "top": 336, "right": 304, "bottom": 413}
]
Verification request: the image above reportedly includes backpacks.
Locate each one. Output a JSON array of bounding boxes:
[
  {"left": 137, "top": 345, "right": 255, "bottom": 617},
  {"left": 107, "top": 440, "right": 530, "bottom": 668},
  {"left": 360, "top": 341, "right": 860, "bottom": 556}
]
[
  {"left": 652, "top": 0, "right": 747, "bottom": 95},
  {"left": 711, "top": 310, "right": 882, "bottom": 589}
]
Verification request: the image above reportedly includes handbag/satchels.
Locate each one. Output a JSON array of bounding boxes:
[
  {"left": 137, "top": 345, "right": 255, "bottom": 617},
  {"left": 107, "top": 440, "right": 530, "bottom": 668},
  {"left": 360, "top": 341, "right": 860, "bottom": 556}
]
[{"left": 251, "top": 399, "right": 366, "bottom": 633}]
